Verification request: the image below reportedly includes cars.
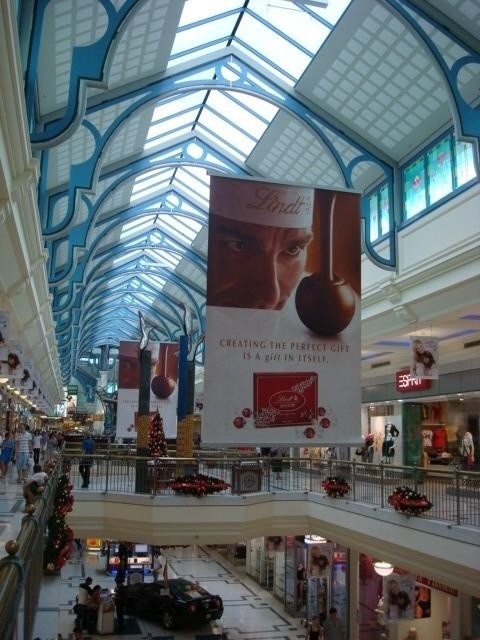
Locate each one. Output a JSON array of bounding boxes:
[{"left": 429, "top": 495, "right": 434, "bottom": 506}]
[{"left": 119, "top": 576, "right": 224, "bottom": 632}]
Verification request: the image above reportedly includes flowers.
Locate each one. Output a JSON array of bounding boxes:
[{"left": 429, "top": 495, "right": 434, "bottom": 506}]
[
  {"left": 42, "top": 469, "right": 78, "bottom": 572},
  {"left": 387, "top": 487, "right": 434, "bottom": 518}
]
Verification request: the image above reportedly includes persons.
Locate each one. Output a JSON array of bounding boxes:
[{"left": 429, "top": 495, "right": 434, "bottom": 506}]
[
  {"left": 387, "top": 580, "right": 401, "bottom": 618},
  {"left": 356, "top": 422, "right": 475, "bottom": 471},
  {"left": 410, "top": 339, "right": 432, "bottom": 378},
  {"left": 310, "top": 546, "right": 322, "bottom": 576},
  {"left": 414, "top": 586, "right": 431, "bottom": 618},
  {"left": 57, "top": 542, "right": 163, "bottom": 639},
  {"left": 373, "top": 591, "right": 389, "bottom": 637},
  {"left": 206, "top": 175, "right": 315, "bottom": 311},
  {"left": 318, "top": 555, "right": 331, "bottom": 576},
  {"left": 305, "top": 616, "right": 325, "bottom": 640},
  {"left": 322, "top": 608, "right": 344, "bottom": 640},
  {"left": 398, "top": 591, "right": 414, "bottom": 618},
  {"left": 0, "top": 423, "right": 64, "bottom": 506},
  {"left": 422, "top": 351, "right": 438, "bottom": 376},
  {"left": 256, "top": 447, "right": 333, "bottom": 480},
  {"left": 79, "top": 430, "right": 96, "bottom": 488},
  {"left": 0, "top": 353, "right": 37, "bottom": 394},
  {"left": 295, "top": 563, "right": 306, "bottom": 612}
]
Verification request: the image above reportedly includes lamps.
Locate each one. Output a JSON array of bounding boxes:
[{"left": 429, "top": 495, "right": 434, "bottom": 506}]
[{"left": 370, "top": 560, "right": 394, "bottom": 578}]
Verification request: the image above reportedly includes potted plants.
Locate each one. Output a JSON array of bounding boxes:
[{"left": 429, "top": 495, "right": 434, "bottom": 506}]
[
  {"left": 322, "top": 476, "right": 352, "bottom": 497},
  {"left": 169, "top": 473, "right": 233, "bottom": 497}
]
